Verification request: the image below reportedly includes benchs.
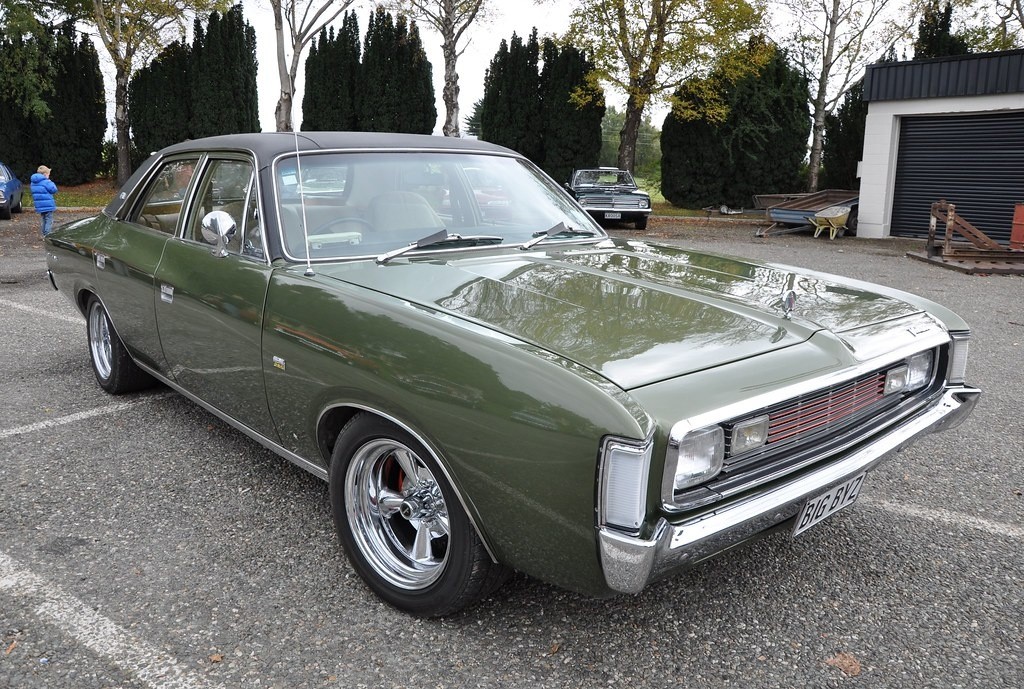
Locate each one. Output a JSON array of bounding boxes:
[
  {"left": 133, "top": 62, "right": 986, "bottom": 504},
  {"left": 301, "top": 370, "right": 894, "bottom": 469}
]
[{"left": 138, "top": 203, "right": 362, "bottom": 245}]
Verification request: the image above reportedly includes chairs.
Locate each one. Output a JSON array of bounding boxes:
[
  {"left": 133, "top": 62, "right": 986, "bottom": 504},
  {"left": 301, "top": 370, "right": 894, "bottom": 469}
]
[
  {"left": 215, "top": 202, "right": 305, "bottom": 261},
  {"left": 368, "top": 191, "right": 447, "bottom": 237}
]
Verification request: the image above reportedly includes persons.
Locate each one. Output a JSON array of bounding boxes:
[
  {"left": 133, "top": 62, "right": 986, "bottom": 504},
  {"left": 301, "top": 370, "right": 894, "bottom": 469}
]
[
  {"left": 30, "top": 165, "right": 58, "bottom": 241},
  {"left": 150, "top": 152, "right": 195, "bottom": 199}
]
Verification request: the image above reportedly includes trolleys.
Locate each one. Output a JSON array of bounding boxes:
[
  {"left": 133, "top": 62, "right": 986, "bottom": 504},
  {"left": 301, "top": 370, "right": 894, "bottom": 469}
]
[{"left": 803, "top": 206, "right": 851, "bottom": 241}]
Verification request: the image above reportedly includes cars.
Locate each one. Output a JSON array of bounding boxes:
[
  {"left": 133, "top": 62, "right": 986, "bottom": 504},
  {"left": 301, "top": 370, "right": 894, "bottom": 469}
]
[
  {"left": 0, "top": 162, "right": 26, "bottom": 220},
  {"left": 563, "top": 166, "right": 652, "bottom": 232},
  {"left": 41, "top": 130, "right": 981, "bottom": 621}
]
